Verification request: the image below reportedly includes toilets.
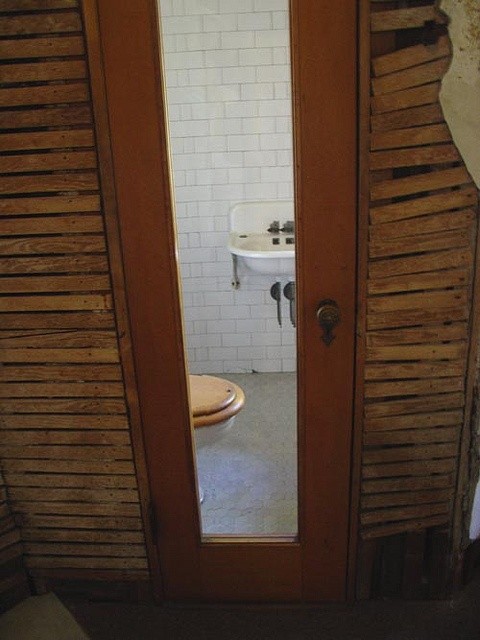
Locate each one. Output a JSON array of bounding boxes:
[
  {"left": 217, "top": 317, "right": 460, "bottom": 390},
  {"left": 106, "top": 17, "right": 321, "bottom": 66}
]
[{"left": 186, "top": 373, "right": 245, "bottom": 504}]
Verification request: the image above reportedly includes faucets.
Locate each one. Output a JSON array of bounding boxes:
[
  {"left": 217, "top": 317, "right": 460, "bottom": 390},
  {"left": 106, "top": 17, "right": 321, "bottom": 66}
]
[{"left": 281, "top": 220, "right": 295, "bottom": 235}]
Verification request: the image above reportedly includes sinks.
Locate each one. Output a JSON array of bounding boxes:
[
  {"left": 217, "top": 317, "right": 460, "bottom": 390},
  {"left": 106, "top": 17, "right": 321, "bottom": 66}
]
[{"left": 227, "top": 235, "right": 296, "bottom": 275}]
